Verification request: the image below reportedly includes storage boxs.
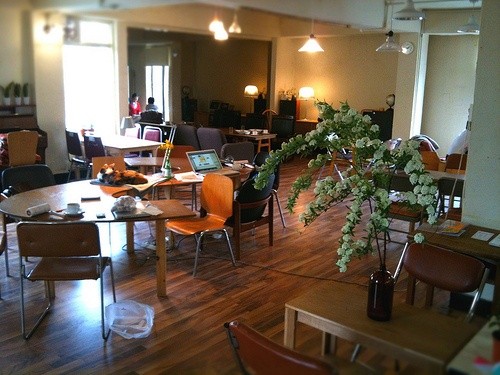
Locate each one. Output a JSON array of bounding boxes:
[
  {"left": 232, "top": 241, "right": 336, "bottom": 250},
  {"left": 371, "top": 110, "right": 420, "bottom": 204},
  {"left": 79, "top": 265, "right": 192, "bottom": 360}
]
[{"left": 273, "top": 114, "right": 294, "bottom": 137}]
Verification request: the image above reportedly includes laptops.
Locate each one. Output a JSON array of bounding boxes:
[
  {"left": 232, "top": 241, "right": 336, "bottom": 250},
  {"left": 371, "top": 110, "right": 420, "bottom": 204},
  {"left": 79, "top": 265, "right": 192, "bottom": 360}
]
[{"left": 185, "top": 149, "right": 241, "bottom": 177}]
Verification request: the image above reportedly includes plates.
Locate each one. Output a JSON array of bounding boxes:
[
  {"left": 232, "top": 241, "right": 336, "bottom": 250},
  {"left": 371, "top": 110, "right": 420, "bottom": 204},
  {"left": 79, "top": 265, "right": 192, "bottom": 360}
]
[
  {"left": 64, "top": 210, "right": 84, "bottom": 217},
  {"left": 250, "top": 128, "right": 262, "bottom": 132},
  {"left": 235, "top": 129, "right": 248, "bottom": 133}
]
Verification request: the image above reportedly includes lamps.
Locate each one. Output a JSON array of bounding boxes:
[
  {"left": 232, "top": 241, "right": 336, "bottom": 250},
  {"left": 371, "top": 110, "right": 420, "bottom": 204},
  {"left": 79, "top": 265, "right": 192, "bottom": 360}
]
[
  {"left": 208, "top": 5, "right": 242, "bottom": 41},
  {"left": 392, "top": 0, "right": 426, "bottom": 20},
  {"left": 298, "top": 86, "right": 316, "bottom": 121},
  {"left": 244, "top": 85, "right": 258, "bottom": 113},
  {"left": 375, "top": 0, "right": 402, "bottom": 52},
  {"left": 298, "top": 7, "right": 325, "bottom": 54},
  {"left": 457, "top": 0, "right": 480, "bottom": 34}
]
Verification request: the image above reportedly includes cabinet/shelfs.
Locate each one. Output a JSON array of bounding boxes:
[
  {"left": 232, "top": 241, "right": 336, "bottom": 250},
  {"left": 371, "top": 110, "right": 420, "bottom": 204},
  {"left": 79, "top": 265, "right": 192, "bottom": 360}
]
[{"left": 0, "top": 104, "right": 35, "bottom": 118}]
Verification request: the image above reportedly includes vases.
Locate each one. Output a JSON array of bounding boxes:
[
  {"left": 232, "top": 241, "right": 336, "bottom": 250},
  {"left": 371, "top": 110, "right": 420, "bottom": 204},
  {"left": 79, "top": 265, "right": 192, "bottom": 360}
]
[
  {"left": 367, "top": 269, "right": 394, "bottom": 322},
  {"left": 165, "top": 164, "right": 172, "bottom": 178}
]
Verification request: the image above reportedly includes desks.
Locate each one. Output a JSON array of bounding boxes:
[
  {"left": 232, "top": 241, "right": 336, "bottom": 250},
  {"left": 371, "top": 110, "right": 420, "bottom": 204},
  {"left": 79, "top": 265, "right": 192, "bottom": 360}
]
[
  {"left": 80, "top": 135, "right": 161, "bottom": 173},
  {"left": 0, "top": 157, "right": 242, "bottom": 297},
  {"left": 407, "top": 219, "right": 500, "bottom": 309},
  {"left": 222, "top": 127, "right": 277, "bottom": 154},
  {"left": 398, "top": 170, "right": 465, "bottom": 186},
  {"left": 284, "top": 277, "right": 478, "bottom": 375}
]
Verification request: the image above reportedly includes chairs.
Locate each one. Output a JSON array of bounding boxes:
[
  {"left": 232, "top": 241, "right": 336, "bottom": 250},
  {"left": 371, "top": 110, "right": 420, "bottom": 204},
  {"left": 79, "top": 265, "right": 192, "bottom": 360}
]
[{"left": 0, "top": 124, "right": 490, "bottom": 375}]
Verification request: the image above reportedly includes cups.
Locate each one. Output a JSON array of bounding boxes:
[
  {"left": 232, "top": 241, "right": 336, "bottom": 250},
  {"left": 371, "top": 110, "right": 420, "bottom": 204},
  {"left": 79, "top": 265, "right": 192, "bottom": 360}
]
[
  {"left": 252, "top": 131, "right": 257, "bottom": 135},
  {"left": 263, "top": 130, "right": 268, "bottom": 134},
  {"left": 68, "top": 203, "right": 79, "bottom": 213},
  {"left": 244, "top": 131, "right": 250, "bottom": 134}
]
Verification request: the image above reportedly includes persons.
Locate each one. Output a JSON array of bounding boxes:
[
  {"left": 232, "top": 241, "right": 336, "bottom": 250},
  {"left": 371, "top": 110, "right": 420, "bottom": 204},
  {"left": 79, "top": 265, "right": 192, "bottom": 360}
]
[
  {"left": 146, "top": 97, "right": 158, "bottom": 110},
  {"left": 181, "top": 85, "right": 199, "bottom": 124},
  {"left": 129, "top": 93, "right": 141, "bottom": 116}
]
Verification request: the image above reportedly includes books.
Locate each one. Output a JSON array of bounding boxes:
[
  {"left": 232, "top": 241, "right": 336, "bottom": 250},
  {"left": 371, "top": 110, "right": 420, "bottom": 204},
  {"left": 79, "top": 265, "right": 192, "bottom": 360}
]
[{"left": 114, "top": 201, "right": 152, "bottom": 219}]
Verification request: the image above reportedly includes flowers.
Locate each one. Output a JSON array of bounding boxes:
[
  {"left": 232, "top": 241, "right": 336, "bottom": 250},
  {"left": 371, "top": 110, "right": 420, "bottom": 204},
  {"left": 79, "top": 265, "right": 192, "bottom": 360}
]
[
  {"left": 160, "top": 139, "right": 175, "bottom": 164},
  {"left": 253, "top": 98, "right": 438, "bottom": 273}
]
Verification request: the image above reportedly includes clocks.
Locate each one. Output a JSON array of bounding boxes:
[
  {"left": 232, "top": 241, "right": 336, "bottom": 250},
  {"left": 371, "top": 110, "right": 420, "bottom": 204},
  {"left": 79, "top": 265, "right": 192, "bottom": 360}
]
[{"left": 401, "top": 41, "right": 416, "bottom": 54}]
